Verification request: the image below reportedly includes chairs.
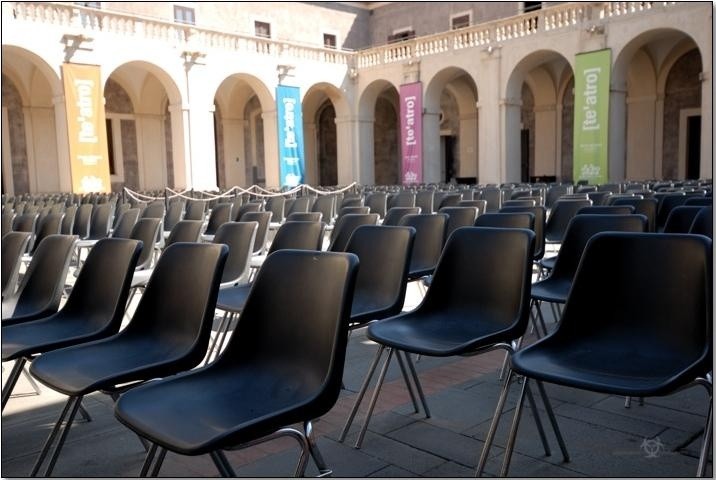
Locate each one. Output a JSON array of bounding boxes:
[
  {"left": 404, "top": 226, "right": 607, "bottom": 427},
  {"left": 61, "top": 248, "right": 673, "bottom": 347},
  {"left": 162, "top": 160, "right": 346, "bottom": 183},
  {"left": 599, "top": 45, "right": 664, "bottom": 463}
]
[
  {"left": 339, "top": 226, "right": 420, "bottom": 444},
  {"left": 499, "top": 233, "right": 713, "bottom": 478},
  {"left": 29, "top": 242, "right": 231, "bottom": 479},
  {"left": 353, "top": 226, "right": 551, "bottom": 477},
  {"left": 113, "top": 250, "right": 359, "bottom": 479}
]
